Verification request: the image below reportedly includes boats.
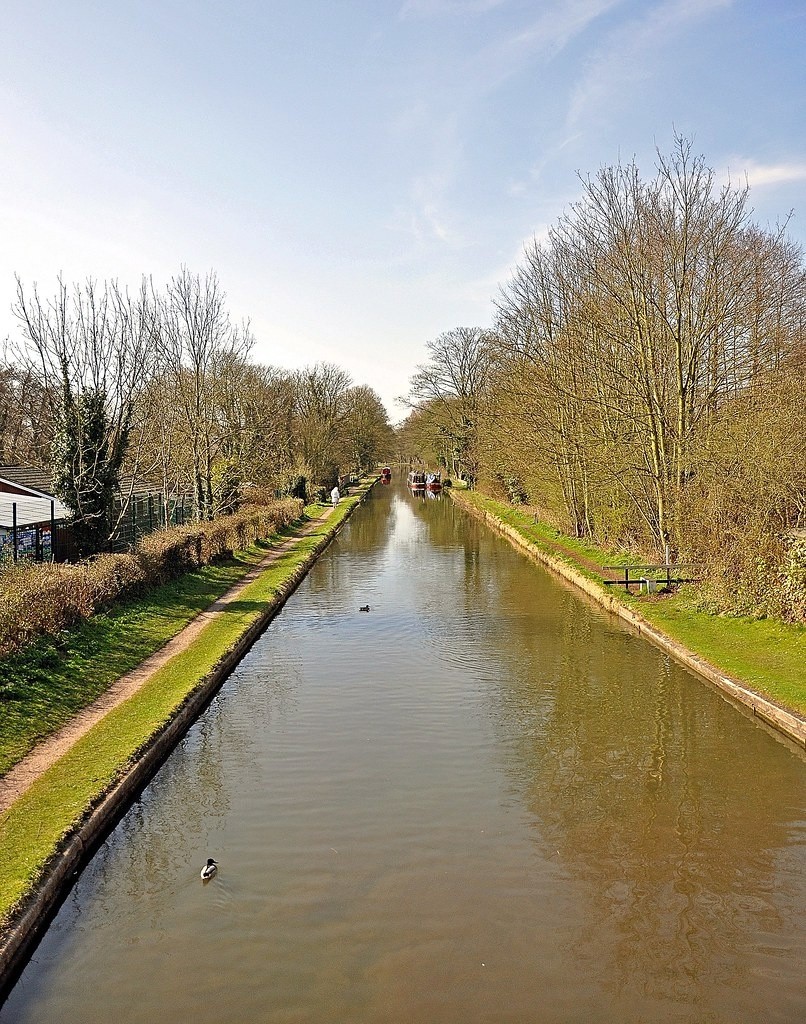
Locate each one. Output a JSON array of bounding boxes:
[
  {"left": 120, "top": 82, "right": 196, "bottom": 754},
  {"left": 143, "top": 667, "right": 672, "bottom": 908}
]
[
  {"left": 381, "top": 466, "right": 392, "bottom": 479},
  {"left": 428, "top": 471, "right": 443, "bottom": 495},
  {"left": 406, "top": 471, "right": 427, "bottom": 491}
]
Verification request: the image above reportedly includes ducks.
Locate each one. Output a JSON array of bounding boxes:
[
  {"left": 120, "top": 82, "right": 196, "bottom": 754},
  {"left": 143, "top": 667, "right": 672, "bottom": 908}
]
[
  {"left": 359, "top": 604, "right": 370, "bottom": 612},
  {"left": 200, "top": 858, "right": 218, "bottom": 879}
]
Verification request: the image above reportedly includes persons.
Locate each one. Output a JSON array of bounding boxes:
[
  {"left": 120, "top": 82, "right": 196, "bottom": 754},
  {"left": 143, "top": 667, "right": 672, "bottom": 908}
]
[
  {"left": 437, "top": 470, "right": 441, "bottom": 479},
  {"left": 331, "top": 487, "right": 339, "bottom": 509}
]
